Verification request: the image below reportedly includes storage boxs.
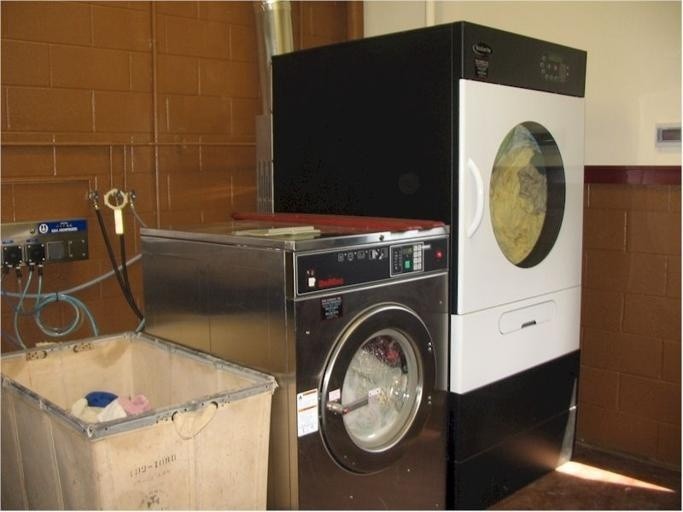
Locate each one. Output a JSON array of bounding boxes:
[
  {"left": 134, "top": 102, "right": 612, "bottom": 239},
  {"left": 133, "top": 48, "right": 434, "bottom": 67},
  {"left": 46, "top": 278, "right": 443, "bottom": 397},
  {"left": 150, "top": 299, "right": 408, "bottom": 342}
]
[{"left": 1, "top": 330, "right": 277, "bottom": 510}]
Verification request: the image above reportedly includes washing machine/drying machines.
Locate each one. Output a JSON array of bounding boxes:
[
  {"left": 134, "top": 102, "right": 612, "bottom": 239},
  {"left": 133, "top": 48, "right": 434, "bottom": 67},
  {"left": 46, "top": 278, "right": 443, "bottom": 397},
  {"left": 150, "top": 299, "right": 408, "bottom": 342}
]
[
  {"left": 270, "top": 20, "right": 588, "bottom": 512},
  {"left": 139, "top": 213, "right": 450, "bottom": 511}
]
